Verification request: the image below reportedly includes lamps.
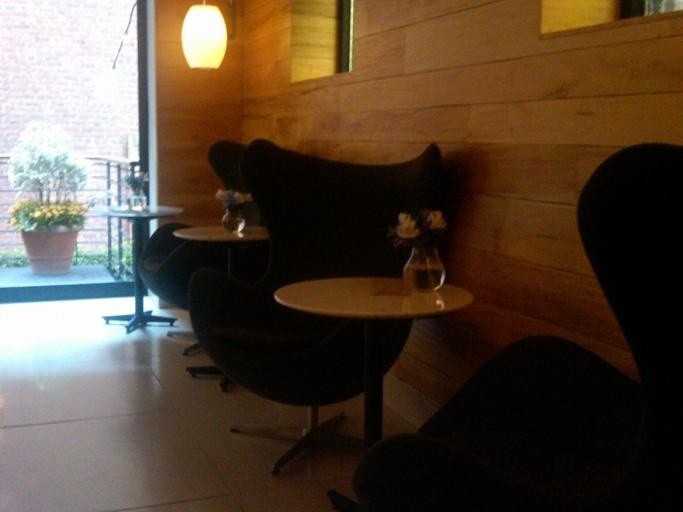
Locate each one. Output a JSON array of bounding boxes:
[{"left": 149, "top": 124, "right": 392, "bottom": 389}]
[{"left": 180, "top": 1, "right": 231, "bottom": 73}]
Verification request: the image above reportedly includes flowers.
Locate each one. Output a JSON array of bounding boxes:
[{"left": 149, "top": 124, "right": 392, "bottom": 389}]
[
  {"left": 381, "top": 206, "right": 447, "bottom": 245},
  {"left": 12, "top": 198, "right": 82, "bottom": 230},
  {"left": 214, "top": 187, "right": 253, "bottom": 206}
]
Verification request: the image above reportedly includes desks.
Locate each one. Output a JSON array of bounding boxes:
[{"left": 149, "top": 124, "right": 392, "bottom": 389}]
[{"left": 273, "top": 275, "right": 474, "bottom": 447}]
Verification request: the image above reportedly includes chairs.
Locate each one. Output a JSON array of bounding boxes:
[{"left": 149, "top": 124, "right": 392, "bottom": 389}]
[
  {"left": 107, "top": 204, "right": 183, "bottom": 331},
  {"left": 137, "top": 140, "right": 265, "bottom": 310},
  {"left": 179, "top": 139, "right": 463, "bottom": 478},
  {"left": 325, "top": 142, "right": 683, "bottom": 512}
]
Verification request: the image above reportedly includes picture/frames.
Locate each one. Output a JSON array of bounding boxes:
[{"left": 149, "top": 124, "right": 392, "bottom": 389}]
[
  {"left": 537, "top": 0, "right": 623, "bottom": 40},
  {"left": 284, "top": 0, "right": 344, "bottom": 85}
]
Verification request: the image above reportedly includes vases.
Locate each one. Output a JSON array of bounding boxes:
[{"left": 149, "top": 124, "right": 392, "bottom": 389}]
[
  {"left": 222, "top": 207, "right": 246, "bottom": 232},
  {"left": 404, "top": 245, "right": 445, "bottom": 293},
  {"left": 23, "top": 230, "right": 77, "bottom": 275}
]
